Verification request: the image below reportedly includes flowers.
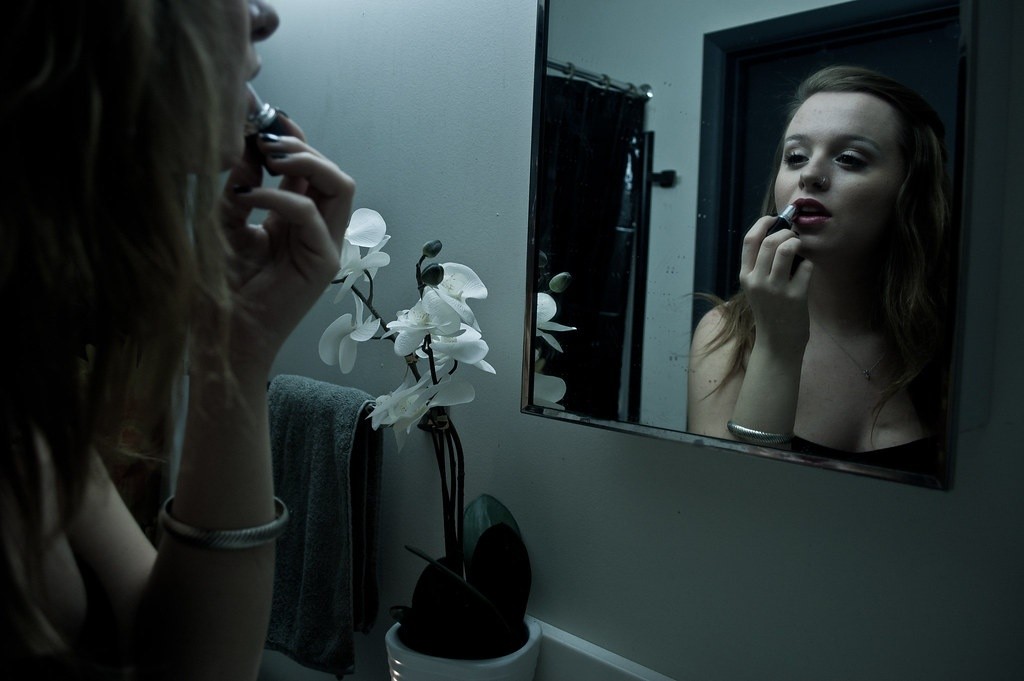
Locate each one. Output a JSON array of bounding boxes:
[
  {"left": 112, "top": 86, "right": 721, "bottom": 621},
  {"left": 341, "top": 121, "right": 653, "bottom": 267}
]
[
  {"left": 530, "top": 246, "right": 572, "bottom": 410},
  {"left": 318, "top": 207, "right": 531, "bottom": 656}
]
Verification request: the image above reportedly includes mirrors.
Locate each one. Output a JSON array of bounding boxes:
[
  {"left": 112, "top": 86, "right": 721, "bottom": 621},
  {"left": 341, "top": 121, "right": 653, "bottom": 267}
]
[{"left": 521, "top": 0, "right": 973, "bottom": 491}]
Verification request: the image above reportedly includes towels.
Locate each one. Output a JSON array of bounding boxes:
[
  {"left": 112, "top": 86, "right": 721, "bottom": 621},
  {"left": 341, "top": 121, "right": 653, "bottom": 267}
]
[{"left": 262, "top": 373, "right": 390, "bottom": 680}]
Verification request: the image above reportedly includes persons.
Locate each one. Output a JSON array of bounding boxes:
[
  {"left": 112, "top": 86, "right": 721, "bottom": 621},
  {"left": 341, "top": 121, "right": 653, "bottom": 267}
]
[
  {"left": 689, "top": 66, "right": 965, "bottom": 485},
  {"left": 0, "top": 0, "right": 357, "bottom": 681}
]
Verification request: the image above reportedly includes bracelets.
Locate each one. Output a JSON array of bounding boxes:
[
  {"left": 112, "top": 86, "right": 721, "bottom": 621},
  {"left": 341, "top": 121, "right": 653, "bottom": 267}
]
[
  {"left": 158, "top": 495, "right": 290, "bottom": 547},
  {"left": 726, "top": 420, "right": 795, "bottom": 446}
]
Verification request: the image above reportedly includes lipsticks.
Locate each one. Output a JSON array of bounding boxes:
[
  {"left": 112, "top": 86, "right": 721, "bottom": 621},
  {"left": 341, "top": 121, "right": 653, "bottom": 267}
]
[
  {"left": 244, "top": 82, "right": 293, "bottom": 160},
  {"left": 766, "top": 204, "right": 797, "bottom": 235}
]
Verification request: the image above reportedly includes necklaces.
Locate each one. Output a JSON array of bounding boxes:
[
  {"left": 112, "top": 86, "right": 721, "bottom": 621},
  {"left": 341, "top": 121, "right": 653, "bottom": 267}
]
[{"left": 815, "top": 320, "right": 890, "bottom": 383}]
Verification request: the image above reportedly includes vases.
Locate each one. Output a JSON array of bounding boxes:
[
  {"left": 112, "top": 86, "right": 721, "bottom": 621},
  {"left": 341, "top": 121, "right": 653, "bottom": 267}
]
[{"left": 385, "top": 616, "right": 542, "bottom": 681}]
